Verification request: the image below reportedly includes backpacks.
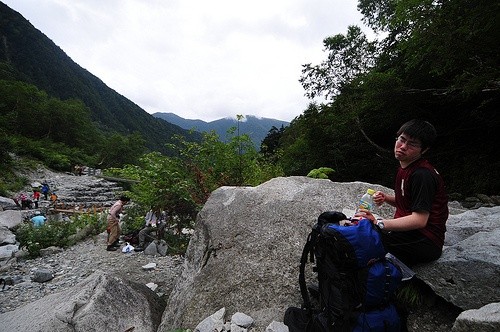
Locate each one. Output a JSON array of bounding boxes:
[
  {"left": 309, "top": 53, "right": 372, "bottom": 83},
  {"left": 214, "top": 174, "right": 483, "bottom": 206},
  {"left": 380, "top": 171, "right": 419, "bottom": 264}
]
[{"left": 298, "top": 227, "right": 365, "bottom": 332}]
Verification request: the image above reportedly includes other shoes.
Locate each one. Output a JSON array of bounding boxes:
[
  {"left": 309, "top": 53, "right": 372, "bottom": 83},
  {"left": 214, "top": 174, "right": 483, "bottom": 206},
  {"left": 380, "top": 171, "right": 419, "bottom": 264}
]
[
  {"left": 107, "top": 246, "right": 116, "bottom": 251},
  {"left": 113, "top": 242, "right": 120, "bottom": 246},
  {"left": 134, "top": 246, "right": 144, "bottom": 251}
]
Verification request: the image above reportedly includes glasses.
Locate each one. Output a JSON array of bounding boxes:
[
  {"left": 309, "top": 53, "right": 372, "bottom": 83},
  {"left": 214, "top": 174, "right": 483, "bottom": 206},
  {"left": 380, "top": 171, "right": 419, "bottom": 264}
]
[{"left": 395, "top": 137, "right": 426, "bottom": 150}]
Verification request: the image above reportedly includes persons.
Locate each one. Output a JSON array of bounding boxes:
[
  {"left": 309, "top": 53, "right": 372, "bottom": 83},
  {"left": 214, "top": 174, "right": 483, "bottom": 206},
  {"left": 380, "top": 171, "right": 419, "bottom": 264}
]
[
  {"left": 30, "top": 210, "right": 47, "bottom": 228},
  {"left": 106, "top": 195, "right": 130, "bottom": 251},
  {"left": 134, "top": 205, "right": 165, "bottom": 251},
  {"left": 307, "top": 120, "right": 450, "bottom": 300},
  {"left": 13, "top": 175, "right": 59, "bottom": 210},
  {"left": 74, "top": 164, "right": 96, "bottom": 176}
]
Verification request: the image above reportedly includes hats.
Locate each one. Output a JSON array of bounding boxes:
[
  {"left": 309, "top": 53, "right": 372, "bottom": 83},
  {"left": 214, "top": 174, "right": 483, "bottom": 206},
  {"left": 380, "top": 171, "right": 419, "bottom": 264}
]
[
  {"left": 34, "top": 211, "right": 41, "bottom": 215},
  {"left": 121, "top": 196, "right": 130, "bottom": 201}
]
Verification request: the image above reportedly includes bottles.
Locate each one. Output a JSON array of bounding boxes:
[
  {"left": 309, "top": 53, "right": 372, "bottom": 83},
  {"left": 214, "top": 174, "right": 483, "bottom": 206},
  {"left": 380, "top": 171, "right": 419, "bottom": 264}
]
[{"left": 358, "top": 189, "right": 375, "bottom": 213}]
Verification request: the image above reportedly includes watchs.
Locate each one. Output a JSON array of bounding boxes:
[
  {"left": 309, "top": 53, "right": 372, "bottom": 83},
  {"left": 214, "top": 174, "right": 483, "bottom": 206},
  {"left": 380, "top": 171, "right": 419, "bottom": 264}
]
[{"left": 376, "top": 218, "right": 385, "bottom": 232}]
